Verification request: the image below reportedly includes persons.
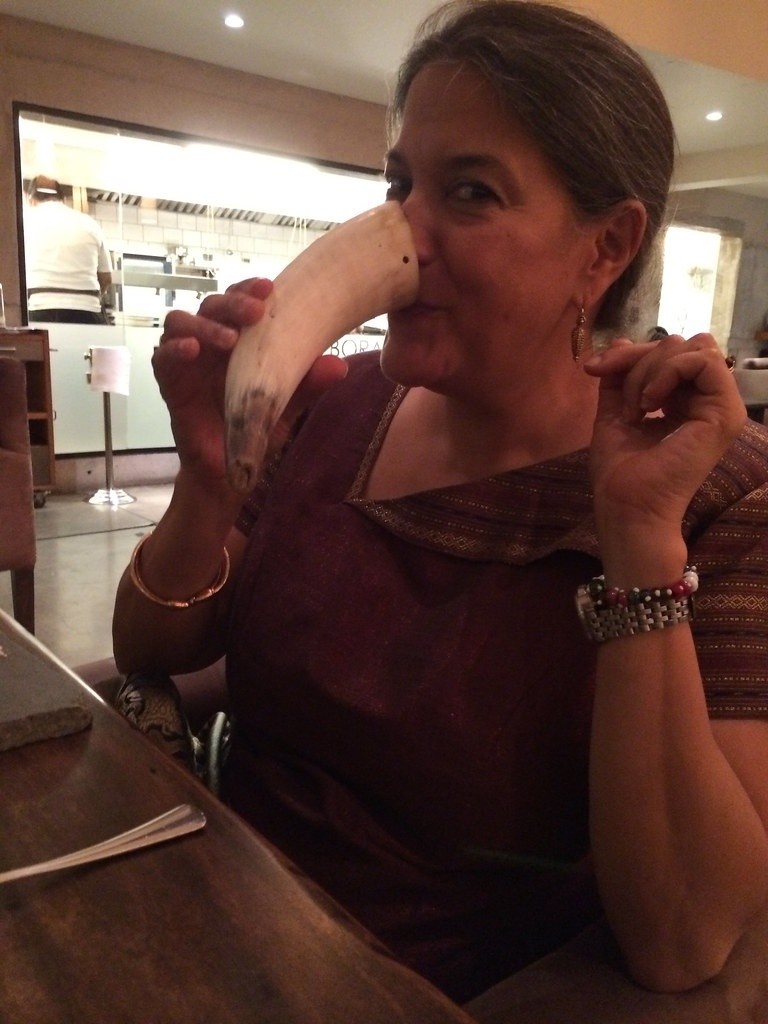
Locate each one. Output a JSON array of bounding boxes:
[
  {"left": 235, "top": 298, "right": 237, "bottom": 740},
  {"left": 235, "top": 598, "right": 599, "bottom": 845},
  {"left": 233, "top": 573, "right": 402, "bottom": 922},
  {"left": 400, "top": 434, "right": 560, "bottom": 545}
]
[
  {"left": 113, "top": 0, "right": 767, "bottom": 991},
  {"left": 21, "top": 174, "right": 111, "bottom": 324}
]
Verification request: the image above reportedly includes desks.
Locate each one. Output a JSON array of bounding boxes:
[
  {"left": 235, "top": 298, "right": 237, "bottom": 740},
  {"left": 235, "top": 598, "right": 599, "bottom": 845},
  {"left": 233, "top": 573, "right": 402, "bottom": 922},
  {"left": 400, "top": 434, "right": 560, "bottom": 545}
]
[{"left": 0, "top": 609, "right": 479, "bottom": 1024}]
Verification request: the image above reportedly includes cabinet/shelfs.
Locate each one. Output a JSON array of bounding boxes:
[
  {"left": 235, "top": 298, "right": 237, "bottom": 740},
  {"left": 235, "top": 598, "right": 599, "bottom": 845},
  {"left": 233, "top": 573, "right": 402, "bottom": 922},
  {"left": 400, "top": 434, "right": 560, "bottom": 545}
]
[{"left": 0, "top": 329, "right": 57, "bottom": 509}]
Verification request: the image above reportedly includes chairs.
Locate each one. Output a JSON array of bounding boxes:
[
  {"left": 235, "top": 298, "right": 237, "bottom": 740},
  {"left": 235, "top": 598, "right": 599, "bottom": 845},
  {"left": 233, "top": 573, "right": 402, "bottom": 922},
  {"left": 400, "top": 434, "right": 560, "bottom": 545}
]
[{"left": 0, "top": 356, "right": 38, "bottom": 634}]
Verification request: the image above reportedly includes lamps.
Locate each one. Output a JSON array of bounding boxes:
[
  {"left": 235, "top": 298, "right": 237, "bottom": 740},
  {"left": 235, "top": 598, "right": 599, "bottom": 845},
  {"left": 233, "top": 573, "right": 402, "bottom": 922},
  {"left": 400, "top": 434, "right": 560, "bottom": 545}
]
[{"left": 83, "top": 344, "right": 137, "bottom": 505}]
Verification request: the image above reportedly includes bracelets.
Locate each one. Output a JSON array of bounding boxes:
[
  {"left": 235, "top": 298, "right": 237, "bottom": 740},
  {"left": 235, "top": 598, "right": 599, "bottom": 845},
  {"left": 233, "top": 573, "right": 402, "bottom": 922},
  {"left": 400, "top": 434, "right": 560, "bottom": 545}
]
[
  {"left": 130, "top": 534, "right": 230, "bottom": 607},
  {"left": 594, "top": 567, "right": 701, "bottom": 608}
]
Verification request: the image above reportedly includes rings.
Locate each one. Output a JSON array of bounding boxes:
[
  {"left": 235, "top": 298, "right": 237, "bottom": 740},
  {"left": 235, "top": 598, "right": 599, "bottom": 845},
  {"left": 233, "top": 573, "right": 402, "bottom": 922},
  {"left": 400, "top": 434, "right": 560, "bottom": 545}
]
[{"left": 725, "top": 356, "right": 737, "bottom": 373}]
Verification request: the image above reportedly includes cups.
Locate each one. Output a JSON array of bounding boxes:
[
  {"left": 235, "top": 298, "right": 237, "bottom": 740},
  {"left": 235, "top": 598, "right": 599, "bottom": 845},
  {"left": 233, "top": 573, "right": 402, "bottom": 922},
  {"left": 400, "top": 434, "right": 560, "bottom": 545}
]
[{"left": 222, "top": 201, "right": 420, "bottom": 497}]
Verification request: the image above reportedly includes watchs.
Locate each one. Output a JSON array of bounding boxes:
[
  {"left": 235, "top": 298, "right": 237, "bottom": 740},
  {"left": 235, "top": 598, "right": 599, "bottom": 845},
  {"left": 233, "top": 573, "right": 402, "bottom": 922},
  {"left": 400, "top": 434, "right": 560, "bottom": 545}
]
[{"left": 574, "top": 582, "right": 697, "bottom": 641}]
